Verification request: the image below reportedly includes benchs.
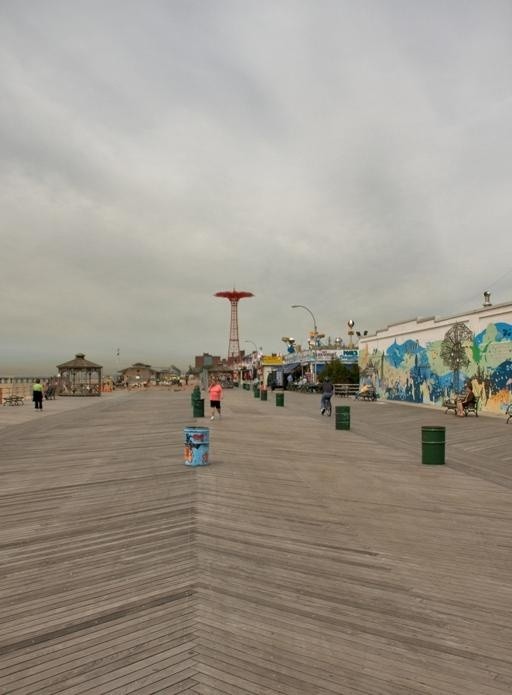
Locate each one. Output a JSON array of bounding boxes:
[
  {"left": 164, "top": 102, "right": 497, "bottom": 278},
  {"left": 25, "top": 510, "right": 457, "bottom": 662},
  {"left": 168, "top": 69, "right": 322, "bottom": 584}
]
[
  {"left": 442, "top": 394, "right": 481, "bottom": 417},
  {"left": 2, "top": 396, "right": 25, "bottom": 406},
  {"left": 505, "top": 404, "right": 512, "bottom": 424}
]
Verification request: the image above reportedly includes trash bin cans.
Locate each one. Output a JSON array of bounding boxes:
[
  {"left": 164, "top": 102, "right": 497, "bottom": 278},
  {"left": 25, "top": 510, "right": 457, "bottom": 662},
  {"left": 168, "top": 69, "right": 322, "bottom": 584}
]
[
  {"left": 421, "top": 426, "right": 446, "bottom": 464},
  {"left": 255, "top": 389, "right": 267, "bottom": 400},
  {"left": 184, "top": 427, "right": 209, "bottom": 466},
  {"left": 192, "top": 386, "right": 204, "bottom": 417},
  {"left": 336, "top": 406, "right": 350, "bottom": 430},
  {"left": 276, "top": 393, "right": 284, "bottom": 406}
]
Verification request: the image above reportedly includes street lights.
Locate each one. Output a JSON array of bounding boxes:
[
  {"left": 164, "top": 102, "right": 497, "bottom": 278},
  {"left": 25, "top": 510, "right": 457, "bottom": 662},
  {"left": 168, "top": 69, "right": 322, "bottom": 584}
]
[
  {"left": 293, "top": 304, "right": 317, "bottom": 382},
  {"left": 245, "top": 340, "right": 259, "bottom": 379}
]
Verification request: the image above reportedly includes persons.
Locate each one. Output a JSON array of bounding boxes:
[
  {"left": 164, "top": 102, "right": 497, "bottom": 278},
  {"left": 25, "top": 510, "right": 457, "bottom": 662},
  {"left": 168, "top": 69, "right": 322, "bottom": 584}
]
[
  {"left": 207, "top": 379, "right": 224, "bottom": 420},
  {"left": 353, "top": 383, "right": 368, "bottom": 401},
  {"left": 315, "top": 379, "right": 320, "bottom": 391},
  {"left": 244, "top": 368, "right": 307, "bottom": 392},
  {"left": 318, "top": 377, "right": 334, "bottom": 414},
  {"left": 458, "top": 386, "right": 475, "bottom": 417},
  {"left": 32, "top": 379, "right": 43, "bottom": 411},
  {"left": 43, "top": 383, "right": 49, "bottom": 400},
  {"left": 163, "top": 375, "right": 181, "bottom": 385}
]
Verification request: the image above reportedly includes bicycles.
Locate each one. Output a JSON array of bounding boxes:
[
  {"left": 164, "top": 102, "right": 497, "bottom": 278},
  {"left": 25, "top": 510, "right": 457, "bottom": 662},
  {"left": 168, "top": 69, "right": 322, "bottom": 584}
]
[{"left": 319, "top": 389, "right": 332, "bottom": 417}]
